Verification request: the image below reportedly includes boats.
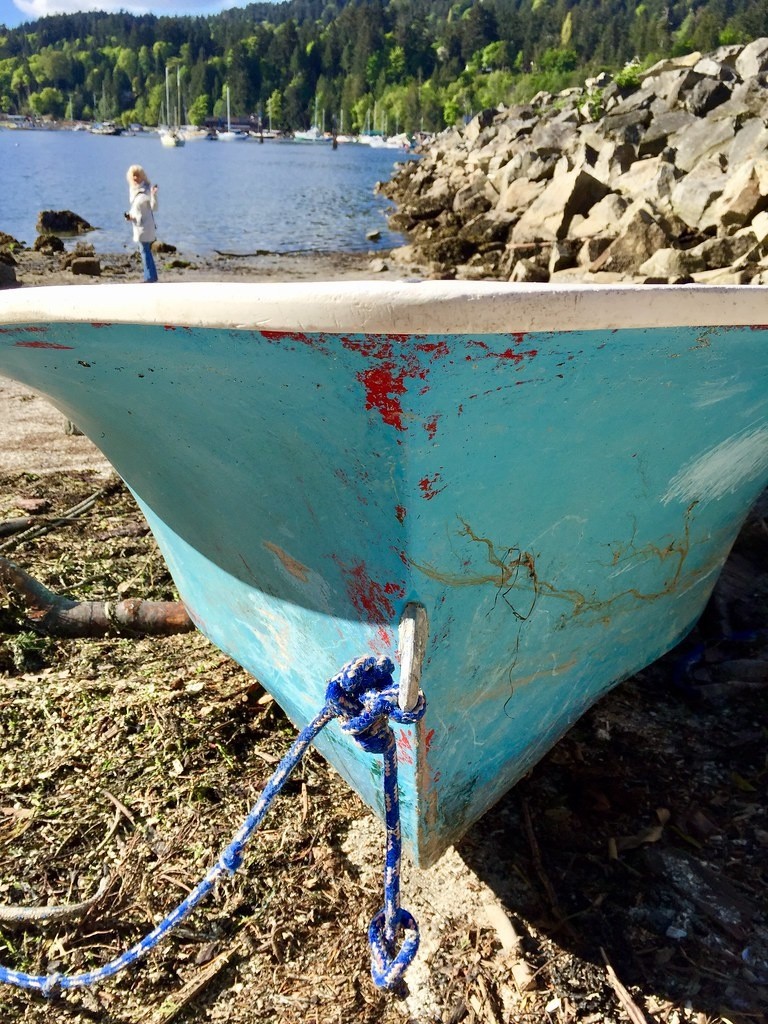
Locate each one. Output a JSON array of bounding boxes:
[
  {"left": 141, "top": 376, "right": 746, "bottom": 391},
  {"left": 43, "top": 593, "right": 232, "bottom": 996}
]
[{"left": 0, "top": 277, "right": 768, "bottom": 873}]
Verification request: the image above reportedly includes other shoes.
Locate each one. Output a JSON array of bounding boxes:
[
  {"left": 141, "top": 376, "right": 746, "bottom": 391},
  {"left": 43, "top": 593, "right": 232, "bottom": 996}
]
[{"left": 140, "top": 279, "right": 154, "bottom": 283}]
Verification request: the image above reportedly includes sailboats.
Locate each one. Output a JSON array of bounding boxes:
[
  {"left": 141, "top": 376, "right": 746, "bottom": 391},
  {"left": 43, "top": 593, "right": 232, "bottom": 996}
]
[{"left": 89, "top": 63, "right": 357, "bottom": 149}]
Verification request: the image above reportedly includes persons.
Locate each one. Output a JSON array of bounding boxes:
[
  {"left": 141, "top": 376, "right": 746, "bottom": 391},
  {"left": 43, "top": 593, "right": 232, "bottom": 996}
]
[{"left": 124, "top": 164, "right": 159, "bottom": 284}]
